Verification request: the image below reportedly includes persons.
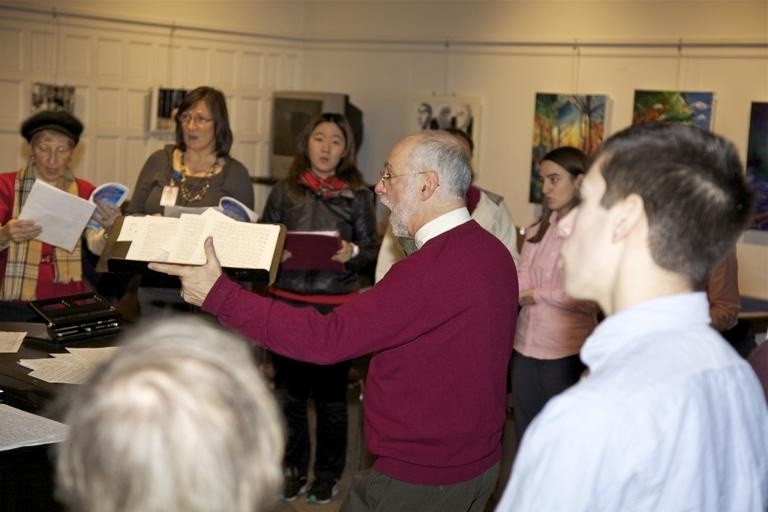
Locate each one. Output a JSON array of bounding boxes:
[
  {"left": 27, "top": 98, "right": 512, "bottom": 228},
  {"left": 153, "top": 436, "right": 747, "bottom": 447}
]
[
  {"left": 0, "top": 109, "right": 123, "bottom": 302},
  {"left": 131, "top": 87, "right": 254, "bottom": 215},
  {"left": 375, "top": 128, "right": 519, "bottom": 284},
  {"left": 515, "top": 145, "right": 601, "bottom": 446},
  {"left": 434, "top": 105, "right": 454, "bottom": 130},
  {"left": 263, "top": 113, "right": 376, "bottom": 503},
  {"left": 701, "top": 243, "right": 742, "bottom": 335},
  {"left": 149, "top": 128, "right": 521, "bottom": 512},
  {"left": 494, "top": 121, "right": 768, "bottom": 512},
  {"left": 452, "top": 105, "right": 472, "bottom": 133},
  {"left": 420, "top": 102, "right": 433, "bottom": 129},
  {"left": 52, "top": 315, "right": 289, "bottom": 511}
]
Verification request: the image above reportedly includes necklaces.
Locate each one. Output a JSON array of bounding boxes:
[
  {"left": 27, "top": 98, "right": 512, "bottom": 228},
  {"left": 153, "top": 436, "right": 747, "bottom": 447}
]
[{"left": 180, "top": 153, "right": 221, "bottom": 202}]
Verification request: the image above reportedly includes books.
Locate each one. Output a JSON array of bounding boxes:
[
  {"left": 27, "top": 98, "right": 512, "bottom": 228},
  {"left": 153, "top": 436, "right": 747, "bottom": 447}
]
[
  {"left": 281, "top": 228, "right": 346, "bottom": 273},
  {"left": 18, "top": 178, "right": 130, "bottom": 252},
  {"left": 125, "top": 212, "right": 281, "bottom": 271},
  {"left": 164, "top": 196, "right": 259, "bottom": 223}
]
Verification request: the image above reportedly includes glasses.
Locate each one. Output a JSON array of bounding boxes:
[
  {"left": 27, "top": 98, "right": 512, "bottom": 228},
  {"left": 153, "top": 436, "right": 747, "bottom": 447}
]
[
  {"left": 175, "top": 111, "right": 215, "bottom": 126},
  {"left": 379, "top": 168, "right": 428, "bottom": 188}
]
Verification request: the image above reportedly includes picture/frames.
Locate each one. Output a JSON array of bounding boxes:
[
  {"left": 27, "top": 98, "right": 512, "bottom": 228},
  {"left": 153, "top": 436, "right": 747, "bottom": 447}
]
[{"left": 403, "top": 95, "right": 481, "bottom": 178}]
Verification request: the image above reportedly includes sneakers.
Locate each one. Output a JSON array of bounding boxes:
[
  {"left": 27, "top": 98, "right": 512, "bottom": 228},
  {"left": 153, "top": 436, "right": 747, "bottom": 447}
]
[
  {"left": 281, "top": 466, "right": 308, "bottom": 502},
  {"left": 304, "top": 478, "right": 341, "bottom": 506}
]
